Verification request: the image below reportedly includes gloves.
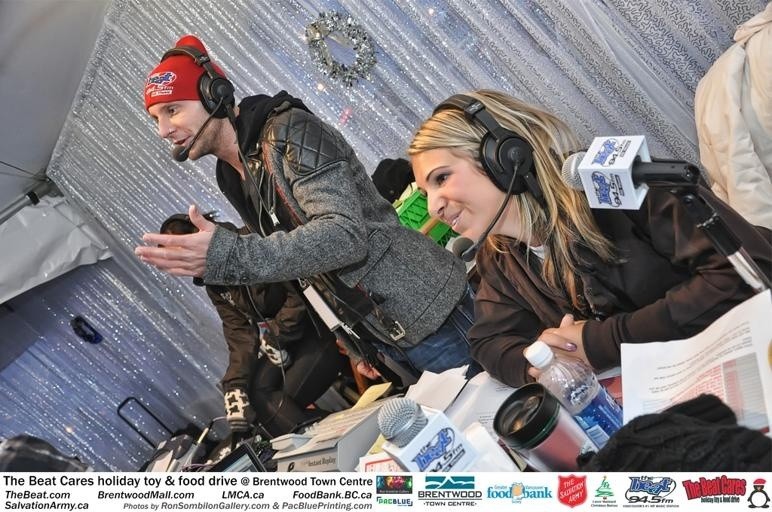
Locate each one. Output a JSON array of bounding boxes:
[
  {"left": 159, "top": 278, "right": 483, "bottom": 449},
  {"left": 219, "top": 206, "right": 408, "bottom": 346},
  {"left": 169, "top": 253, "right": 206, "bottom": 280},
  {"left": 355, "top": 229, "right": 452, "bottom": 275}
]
[
  {"left": 261, "top": 337, "right": 292, "bottom": 370},
  {"left": 224, "top": 382, "right": 257, "bottom": 434}
]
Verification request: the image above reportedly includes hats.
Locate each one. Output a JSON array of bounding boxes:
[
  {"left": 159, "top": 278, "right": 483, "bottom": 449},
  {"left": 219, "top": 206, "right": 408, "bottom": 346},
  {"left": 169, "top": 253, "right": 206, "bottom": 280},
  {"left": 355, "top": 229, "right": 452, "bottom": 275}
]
[{"left": 144, "top": 35, "right": 227, "bottom": 114}]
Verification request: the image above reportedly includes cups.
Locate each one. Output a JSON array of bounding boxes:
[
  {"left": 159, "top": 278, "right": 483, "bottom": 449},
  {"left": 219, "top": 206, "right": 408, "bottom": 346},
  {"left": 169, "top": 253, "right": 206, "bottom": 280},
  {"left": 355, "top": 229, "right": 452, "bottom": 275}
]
[{"left": 492, "top": 381, "right": 599, "bottom": 472}]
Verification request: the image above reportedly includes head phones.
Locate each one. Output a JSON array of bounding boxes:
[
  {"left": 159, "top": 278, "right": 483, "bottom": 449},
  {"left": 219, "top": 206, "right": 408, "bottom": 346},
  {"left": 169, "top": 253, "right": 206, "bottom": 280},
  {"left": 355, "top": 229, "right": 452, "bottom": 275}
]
[
  {"left": 430, "top": 94, "right": 537, "bottom": 194},
  {"left": 159, "top": 46, "right": 235, "bottom": 118}
]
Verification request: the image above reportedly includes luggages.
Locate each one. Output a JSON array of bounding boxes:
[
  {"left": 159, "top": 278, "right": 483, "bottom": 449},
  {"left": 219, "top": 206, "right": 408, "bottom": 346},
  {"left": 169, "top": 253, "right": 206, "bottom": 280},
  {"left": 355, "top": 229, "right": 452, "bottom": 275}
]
[{"left": 117, "top": 396, "right": 220, "bottom": 448}]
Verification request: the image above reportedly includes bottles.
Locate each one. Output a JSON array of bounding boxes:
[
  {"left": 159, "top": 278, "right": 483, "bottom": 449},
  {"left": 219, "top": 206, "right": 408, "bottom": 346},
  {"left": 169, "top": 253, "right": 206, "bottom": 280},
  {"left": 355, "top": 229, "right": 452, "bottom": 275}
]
[{"left": 522, "top": 340, "right": 623, "bottom": 452}]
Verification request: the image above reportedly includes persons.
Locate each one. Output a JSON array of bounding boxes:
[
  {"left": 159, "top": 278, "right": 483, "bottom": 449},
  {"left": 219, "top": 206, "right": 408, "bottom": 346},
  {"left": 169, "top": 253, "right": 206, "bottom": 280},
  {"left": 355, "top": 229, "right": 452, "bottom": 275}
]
[
  {"left": 402, "top": 82, "right": 772, "bottom": 389},
  {"left": 155, "top": 211, "right": 347, "bottom": 437},
  {"left": 131, "top": 34, "right": 491, "bottom": 382}
]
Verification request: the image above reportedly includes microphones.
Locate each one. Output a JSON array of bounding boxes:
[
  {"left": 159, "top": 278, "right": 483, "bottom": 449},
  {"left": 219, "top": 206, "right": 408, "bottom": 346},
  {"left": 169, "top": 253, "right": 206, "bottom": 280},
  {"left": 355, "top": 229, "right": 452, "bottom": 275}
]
[
  {"left": 451, "top": 168, "right": 530, "bottom": 264},
  {"left": 171, "top": 97, "right": 222, "bottom": 161},
  {"left": 560, "top": 136, "right": 696, "bottom": 211},
  {"left": 376, "top": 395, "right": 478, "bottom": 473}
]
